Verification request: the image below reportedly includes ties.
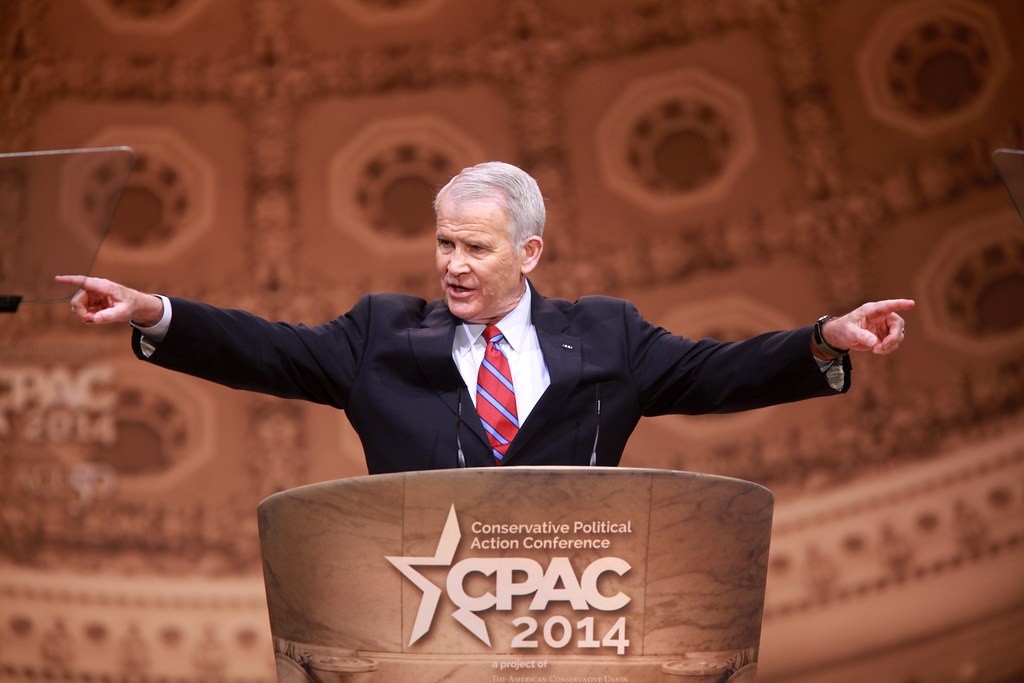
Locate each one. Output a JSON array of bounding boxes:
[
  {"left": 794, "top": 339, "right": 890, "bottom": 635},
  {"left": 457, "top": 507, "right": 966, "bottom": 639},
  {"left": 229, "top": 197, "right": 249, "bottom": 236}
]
[{"left": 476, "top": 325, "right": 520, "bottom": 467}]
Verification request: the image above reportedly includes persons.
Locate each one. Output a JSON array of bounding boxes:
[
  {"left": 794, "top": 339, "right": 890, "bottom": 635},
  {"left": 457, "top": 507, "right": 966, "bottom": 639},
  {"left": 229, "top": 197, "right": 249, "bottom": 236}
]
[{"left": 56, "top": 160, "right": 916, "bottom": 475}]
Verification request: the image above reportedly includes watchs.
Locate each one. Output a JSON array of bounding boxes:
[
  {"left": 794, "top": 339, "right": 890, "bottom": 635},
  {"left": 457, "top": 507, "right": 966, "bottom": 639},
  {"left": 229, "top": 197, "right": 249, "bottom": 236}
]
[{"left": 813, "top": 314, "right": 850, "bottom": 360}]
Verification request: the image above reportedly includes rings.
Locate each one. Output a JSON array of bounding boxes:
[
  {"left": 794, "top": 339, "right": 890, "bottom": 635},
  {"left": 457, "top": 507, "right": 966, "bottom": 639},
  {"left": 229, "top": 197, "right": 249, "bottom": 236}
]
[{"left": 901, "top": 329, "right": 904, "bottom": 334}]
